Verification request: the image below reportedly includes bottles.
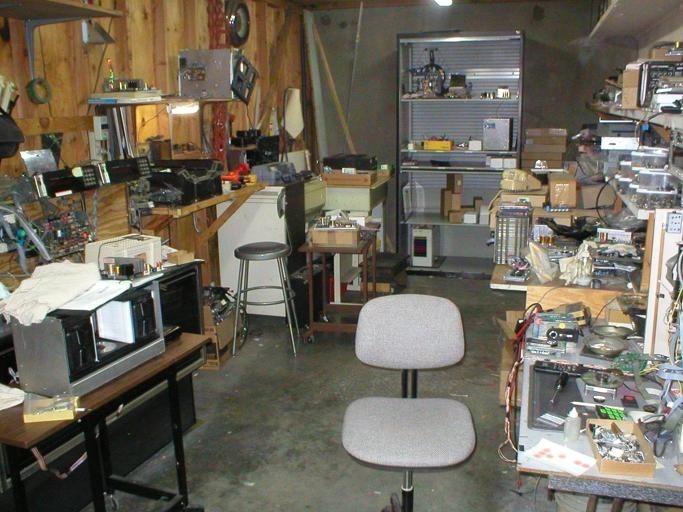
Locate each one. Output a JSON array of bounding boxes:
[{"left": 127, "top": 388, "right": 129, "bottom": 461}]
[
  {"left": 103, "top": 58, "right": 114, "bottom": 91},
  {"left": 578, "top": 244, "right": 593, "bottom": 285}
]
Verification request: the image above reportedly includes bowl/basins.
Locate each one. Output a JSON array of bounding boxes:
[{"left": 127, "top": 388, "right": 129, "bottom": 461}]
[{"left": 583, "top": 326, "right": 633, "bottom": 358}]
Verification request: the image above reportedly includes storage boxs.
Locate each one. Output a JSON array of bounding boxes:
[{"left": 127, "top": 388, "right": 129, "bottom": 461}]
[
  {"left": 500, "top": 126, "right": 580, "bottom": 209},
  {"left": 196, "top": 304, "right": 244, "bottom": 372},
  {"left": 439, "top": 173, "right": 500, "bottom": 230}
]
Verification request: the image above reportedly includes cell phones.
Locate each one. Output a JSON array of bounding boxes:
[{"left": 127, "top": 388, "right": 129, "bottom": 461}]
[{"left": 534, "top": 360, "right": 582, "bottom": 377}]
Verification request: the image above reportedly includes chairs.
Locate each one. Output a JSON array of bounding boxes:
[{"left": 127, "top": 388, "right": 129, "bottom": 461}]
[{"left": 340, "top": 293, "right": 477, "bottom": 512}]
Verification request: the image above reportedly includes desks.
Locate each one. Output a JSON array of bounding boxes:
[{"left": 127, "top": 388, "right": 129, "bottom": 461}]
[
  {"left": 516, "top": 322, "right": 683, "bottom": 512},
  {"left": 215, "top": 169, "right": 393, "bottom": 347},
  {"left": 491, "top": 237, "right": 650, "bottom": 326},
  {"left": 0, "top": 332, "right": 212, "bottom": 512}
]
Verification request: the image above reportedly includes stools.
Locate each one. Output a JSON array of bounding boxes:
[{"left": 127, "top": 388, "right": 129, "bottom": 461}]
[{"left": 230, "top": 241, "right": 301, "bottom": 360}]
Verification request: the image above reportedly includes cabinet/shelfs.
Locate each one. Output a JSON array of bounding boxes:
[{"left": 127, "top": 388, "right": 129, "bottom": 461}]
[
  {"left": 393, "top": 27, "right": 524, "bottom": 280},
  {"left": 589, "top": 0, "right": 683, "bottom": 222}
]
